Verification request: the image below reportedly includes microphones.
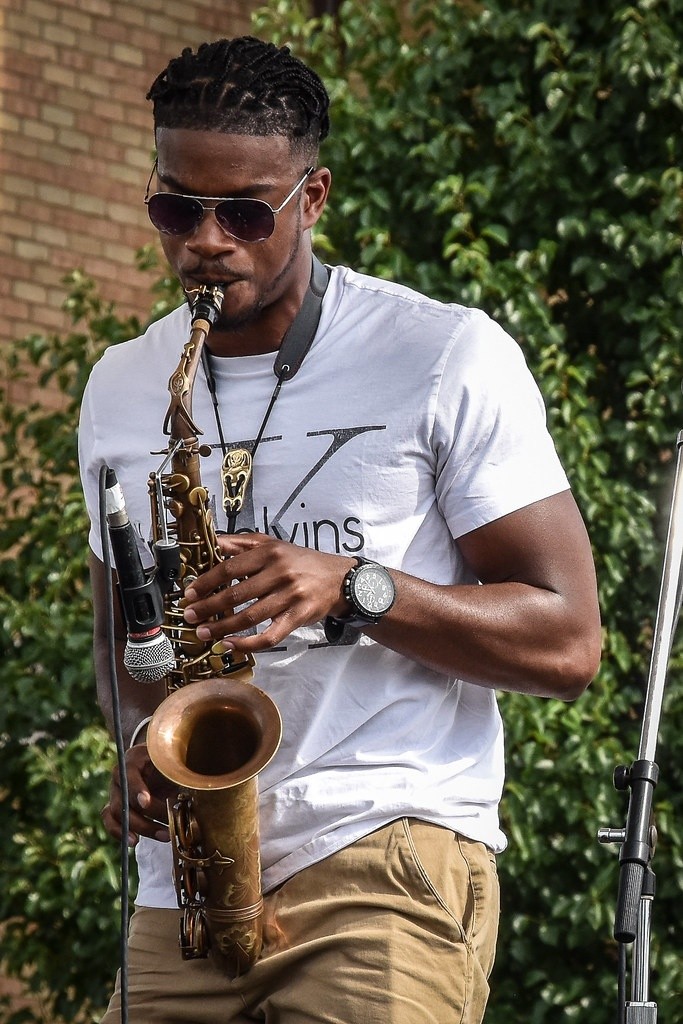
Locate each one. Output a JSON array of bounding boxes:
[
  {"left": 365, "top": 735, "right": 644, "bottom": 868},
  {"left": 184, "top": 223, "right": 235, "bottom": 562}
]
[{"left": 102, "top": 468, "right": 177, "bottom": 684}]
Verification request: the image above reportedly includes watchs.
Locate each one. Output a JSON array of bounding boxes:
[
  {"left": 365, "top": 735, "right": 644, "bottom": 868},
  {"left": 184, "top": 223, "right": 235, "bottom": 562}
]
[{"left": 308, "top": 556, "right": 396, "bottom": 651}]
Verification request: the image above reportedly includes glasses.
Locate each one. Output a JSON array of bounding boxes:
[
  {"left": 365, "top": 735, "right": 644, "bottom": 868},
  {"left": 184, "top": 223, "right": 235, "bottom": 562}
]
[{"left": 145, "top": 158, "right": 313, "bottom": 243}]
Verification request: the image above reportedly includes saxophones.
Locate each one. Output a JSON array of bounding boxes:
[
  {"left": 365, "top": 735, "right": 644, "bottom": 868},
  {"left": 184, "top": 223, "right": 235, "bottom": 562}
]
[{"left": 142, "top": 281, "right": 286, "bottom": 983}]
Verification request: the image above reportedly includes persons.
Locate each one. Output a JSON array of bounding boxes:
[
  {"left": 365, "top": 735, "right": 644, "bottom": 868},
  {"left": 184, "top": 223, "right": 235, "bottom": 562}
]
[{"left": 78, "top": 38, "right": 603, "bottom": 1024}]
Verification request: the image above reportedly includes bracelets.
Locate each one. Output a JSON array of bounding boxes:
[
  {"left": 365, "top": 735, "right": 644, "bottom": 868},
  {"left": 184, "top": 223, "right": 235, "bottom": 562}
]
[{"left": 129, "top": 716, "right": 153, "bottom": 751}]
